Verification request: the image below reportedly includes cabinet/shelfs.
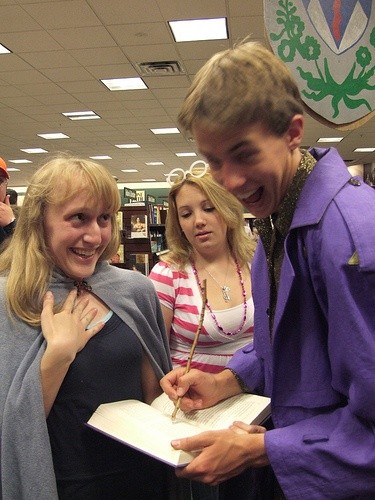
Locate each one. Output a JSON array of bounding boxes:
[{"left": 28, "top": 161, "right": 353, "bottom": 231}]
[{"left": 110, "top": 204, "right": 168, "bottom": 276}]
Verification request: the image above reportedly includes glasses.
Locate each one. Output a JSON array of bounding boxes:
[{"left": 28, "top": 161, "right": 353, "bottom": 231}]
[
  {"left": 0, "top": 177, "right": 7, "bottom": 187},
  {"left": 166, "top": 160, "right": 210, "bottom": 186}
]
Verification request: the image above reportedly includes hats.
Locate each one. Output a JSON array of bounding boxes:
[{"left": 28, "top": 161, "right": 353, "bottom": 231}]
[{"left": 0, "top": 158, "right": 9, "bottom": 179}]
